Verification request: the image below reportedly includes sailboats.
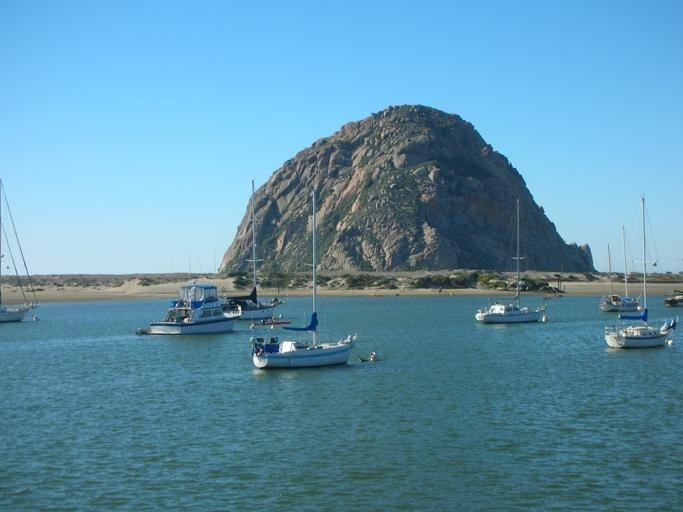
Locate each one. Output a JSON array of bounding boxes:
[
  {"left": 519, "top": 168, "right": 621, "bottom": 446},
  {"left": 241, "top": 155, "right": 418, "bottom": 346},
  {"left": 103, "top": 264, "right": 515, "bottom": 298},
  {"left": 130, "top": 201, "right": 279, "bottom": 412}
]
[
  {"left": 595, "top": 193, "right": 682, "bottom": 348},
  {"left": 0, "top": 179, "right": 39, "bottom": 326},
  {"left": 472, "top": 197, "right": 547, "bottom": 324}
]
